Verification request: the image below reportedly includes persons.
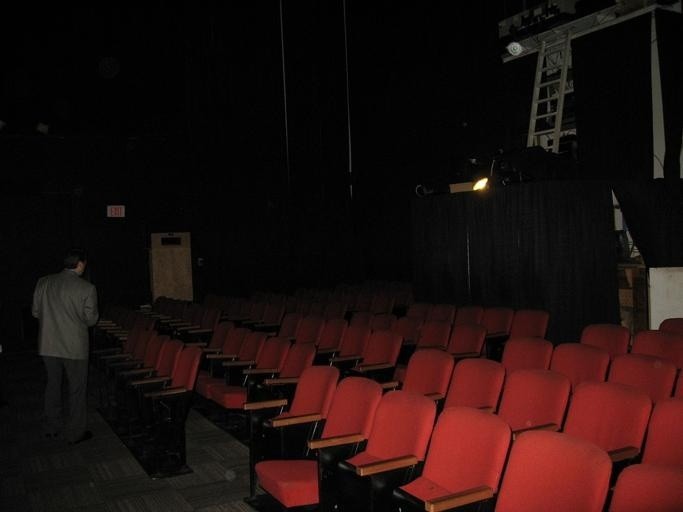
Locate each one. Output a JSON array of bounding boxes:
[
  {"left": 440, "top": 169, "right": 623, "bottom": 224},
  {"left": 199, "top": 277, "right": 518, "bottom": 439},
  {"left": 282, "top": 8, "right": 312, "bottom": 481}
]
[{"left": 31, "top": 249, "right": 98, "bottom": 442}]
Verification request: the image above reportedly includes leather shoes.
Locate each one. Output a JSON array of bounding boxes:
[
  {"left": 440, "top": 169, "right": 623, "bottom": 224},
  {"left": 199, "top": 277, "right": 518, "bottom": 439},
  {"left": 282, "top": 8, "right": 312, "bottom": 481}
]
[
  {"left": 43, "top": 431, "right": 66, "bottom": 446},
  {"left": 69, "top": 429, "right": 97, "bottom": 445}
]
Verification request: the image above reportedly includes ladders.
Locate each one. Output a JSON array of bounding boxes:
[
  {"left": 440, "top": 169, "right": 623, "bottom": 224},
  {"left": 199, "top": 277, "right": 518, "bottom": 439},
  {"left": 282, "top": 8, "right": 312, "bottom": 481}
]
[{"left": 528, "top": 31, "right": 571, "bottom": 154}]
